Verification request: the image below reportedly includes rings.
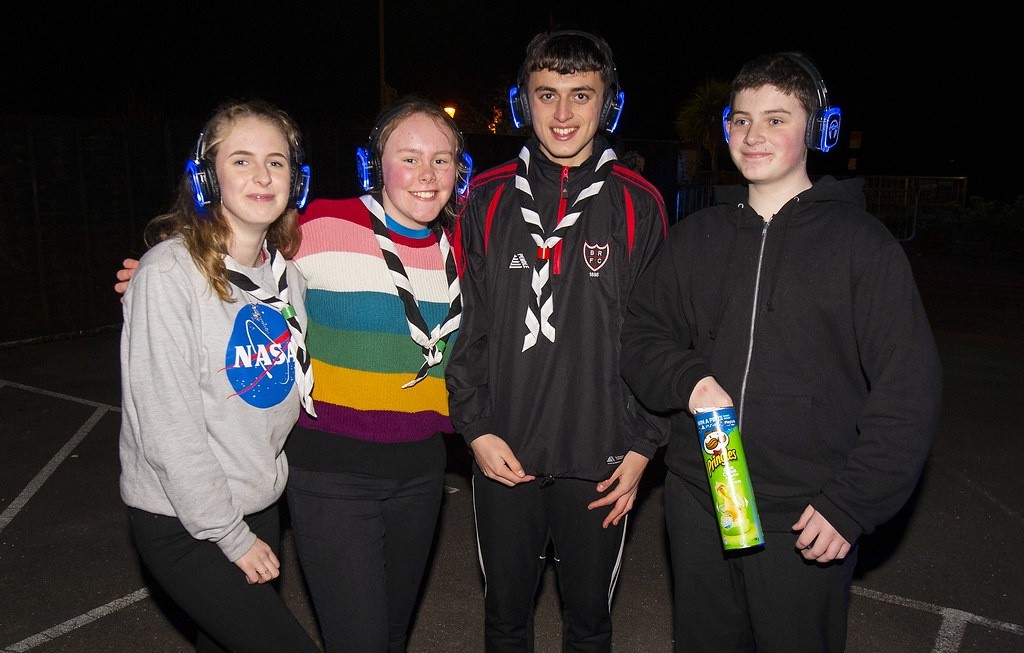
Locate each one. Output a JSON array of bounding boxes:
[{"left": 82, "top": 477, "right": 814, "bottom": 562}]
[{"left": 260, "top": 569, "right": 269, "bottom": 575}]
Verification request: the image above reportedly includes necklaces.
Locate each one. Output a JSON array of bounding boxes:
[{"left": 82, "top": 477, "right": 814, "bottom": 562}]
[{"left": 227, "top": 252, "right": 264, "bottom": 319}]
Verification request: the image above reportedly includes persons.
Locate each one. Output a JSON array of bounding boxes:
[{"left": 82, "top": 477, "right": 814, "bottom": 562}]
[
  {"left": 616, "top": 50, "right": 944, "bottom": 653},
  {"left": 453, "top": 31, "right": 670, "bottom": 653},
  {"left": 112, "top": 100, "right": 464, "bottom": 653},
  {"left": 121, "top": 102, "right": 319, "bottom": 653}
]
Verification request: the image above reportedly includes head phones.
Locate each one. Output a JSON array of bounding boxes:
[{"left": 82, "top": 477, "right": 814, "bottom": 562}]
[
  {"left": 184, "top": 105, "right": 311, "bottom": 211},
  {"left": 508, "top": 31, "right": 624, "bottom": 133},
  {"left": 721, "top": 54, "right": 842, "bottom": 152},
  {"left": 356, "top": 100, "right": 473, "bottom": 196}
]
